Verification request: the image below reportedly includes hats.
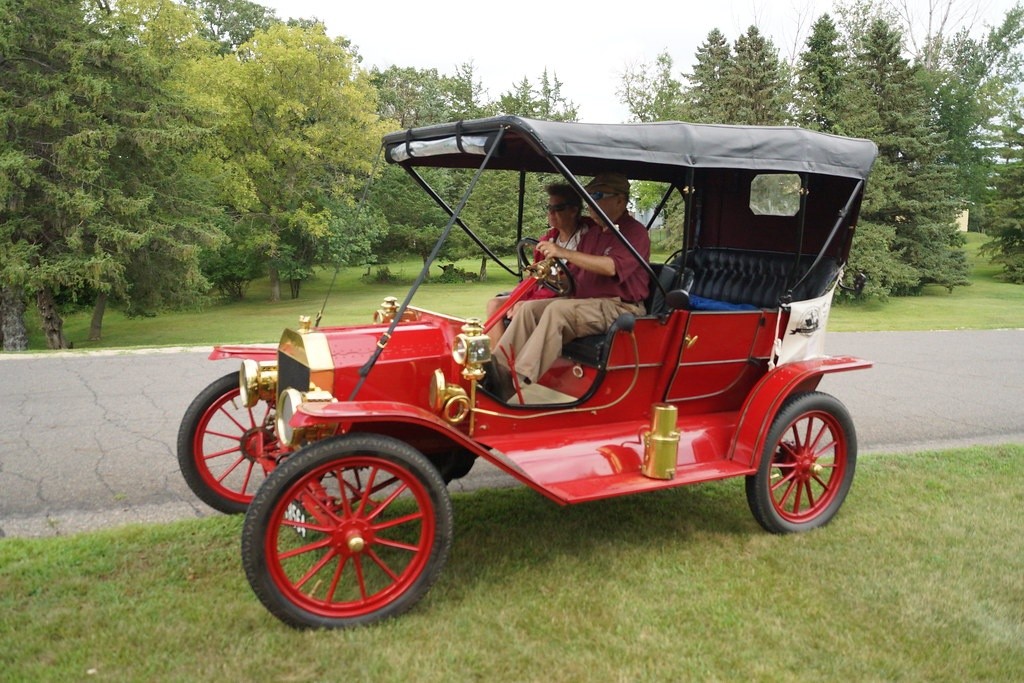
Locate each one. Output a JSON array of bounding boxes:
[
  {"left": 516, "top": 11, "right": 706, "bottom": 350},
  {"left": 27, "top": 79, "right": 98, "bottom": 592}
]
[{"left": 584, "top": 172, "right": 631, "bottom": 195}]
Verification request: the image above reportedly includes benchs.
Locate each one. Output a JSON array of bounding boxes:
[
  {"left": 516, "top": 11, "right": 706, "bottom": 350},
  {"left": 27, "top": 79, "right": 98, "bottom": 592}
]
[
  {"left": 665, "top": 246, "right": 842, "bottom": 311},
  {"left": 502, "top": 264, "right": 679, "bottom": 366}
]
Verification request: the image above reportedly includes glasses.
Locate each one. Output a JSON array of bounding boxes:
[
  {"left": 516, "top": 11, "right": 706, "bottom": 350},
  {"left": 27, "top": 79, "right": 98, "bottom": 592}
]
[
  {"left": 547, "top": 204, "right": 572, "bottom": 212},
  {"left": 591, "top": 192, "right": 616, "bottom": 201}
]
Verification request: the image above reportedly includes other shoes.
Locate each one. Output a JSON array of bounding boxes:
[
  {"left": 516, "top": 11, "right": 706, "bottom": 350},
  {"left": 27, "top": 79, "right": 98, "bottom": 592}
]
[{"left": 482, "top": 354, "right": 517, "bottom": 403}]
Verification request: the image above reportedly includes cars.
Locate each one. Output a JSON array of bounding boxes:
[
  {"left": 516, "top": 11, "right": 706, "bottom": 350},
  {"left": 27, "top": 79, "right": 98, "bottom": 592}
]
[{"left": 174, "top": 114, "right": 874, "bottom": 633}]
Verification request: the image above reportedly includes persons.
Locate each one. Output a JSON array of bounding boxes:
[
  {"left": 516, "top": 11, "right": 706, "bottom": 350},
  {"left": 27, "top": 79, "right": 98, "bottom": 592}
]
[
  {"left": 486, "top": 183, "right": 598, "bottom": 354},
  {"left": 477, "top": 172, "right": 653, "bottom": 402}
]
